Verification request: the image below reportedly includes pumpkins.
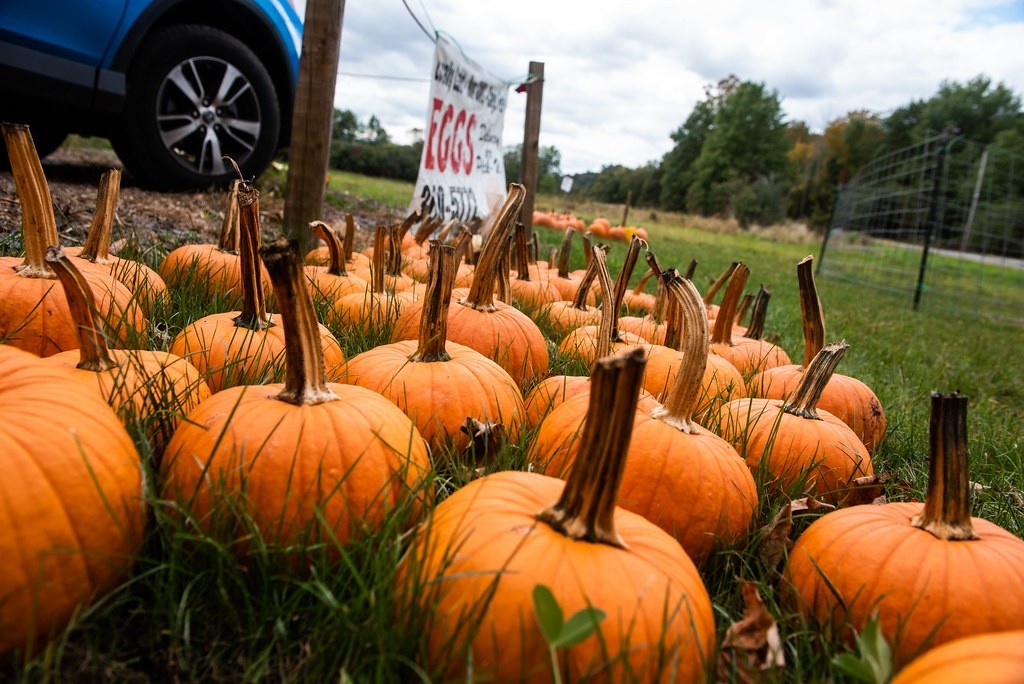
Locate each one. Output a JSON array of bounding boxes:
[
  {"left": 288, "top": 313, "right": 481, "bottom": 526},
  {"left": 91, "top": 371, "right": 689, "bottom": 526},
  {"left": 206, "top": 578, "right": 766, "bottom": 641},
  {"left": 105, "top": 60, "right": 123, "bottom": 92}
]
[{"left": 3, "top": 125, "right": 1024, "bottom": 683}]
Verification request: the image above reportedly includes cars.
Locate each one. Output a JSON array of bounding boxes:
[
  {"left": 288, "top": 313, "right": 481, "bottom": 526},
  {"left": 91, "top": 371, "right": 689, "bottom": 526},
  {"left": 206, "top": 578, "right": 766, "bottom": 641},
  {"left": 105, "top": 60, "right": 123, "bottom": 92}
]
[{"left": 0, "top": 1, "right": 304, "bottom": 195}]
